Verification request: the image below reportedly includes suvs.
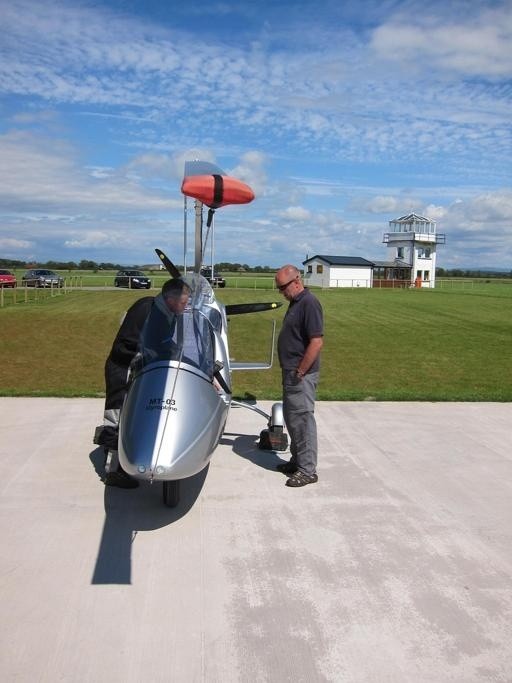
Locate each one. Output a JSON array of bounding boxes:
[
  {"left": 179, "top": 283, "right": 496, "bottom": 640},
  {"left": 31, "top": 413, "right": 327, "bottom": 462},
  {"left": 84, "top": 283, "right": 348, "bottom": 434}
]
[{"left": 200, "top": 269, "right": 225, "bottom": 288}]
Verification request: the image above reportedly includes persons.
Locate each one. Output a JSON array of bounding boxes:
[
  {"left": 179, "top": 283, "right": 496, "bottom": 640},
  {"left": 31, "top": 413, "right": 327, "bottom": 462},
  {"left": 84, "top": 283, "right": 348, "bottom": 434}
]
[
  {"left": 273, "top": 264, "right": 324, "bottom": 487},
  {"left": 101, "top": 278, "right": 192, "bottom": 488}
]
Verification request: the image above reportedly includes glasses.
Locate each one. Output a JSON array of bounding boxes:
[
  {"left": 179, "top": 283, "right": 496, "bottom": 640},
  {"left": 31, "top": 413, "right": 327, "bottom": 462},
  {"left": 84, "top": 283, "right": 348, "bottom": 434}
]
[{"left": 276, "top": 275, "right": 299, "bottom": 291}]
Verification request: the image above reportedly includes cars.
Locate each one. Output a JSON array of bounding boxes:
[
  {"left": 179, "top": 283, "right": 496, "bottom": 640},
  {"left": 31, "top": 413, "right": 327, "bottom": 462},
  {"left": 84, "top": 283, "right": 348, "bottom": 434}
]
[
  {"left": 22, "top": 269, "right": 65, "bottom": 289},
  {"left": 114, "top": 270, "right": 151, "bottom": 289},
  {"left": 0, "top": 270, "right": 17, "bottom": 288}
]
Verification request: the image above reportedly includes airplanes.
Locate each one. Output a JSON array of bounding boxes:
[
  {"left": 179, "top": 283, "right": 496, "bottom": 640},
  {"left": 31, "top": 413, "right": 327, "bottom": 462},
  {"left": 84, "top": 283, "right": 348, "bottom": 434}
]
[{"left": 92, "top": 157, "right": 289, "bottom": 508}]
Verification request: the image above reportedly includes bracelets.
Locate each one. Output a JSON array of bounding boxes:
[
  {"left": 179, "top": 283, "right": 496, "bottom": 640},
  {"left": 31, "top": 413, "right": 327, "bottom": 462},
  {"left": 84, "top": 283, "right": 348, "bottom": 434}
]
[{"left": 295, "top": 368, "right": 304, "bottom": 377}]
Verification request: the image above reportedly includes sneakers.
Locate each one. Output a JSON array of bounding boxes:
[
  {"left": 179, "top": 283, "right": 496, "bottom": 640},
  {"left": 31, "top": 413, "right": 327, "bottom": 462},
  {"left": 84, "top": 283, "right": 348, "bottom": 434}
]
[
  {"left": 276, "top": 462, "right": 297, "bottom": 473},
  {"left": 104, "top": 471, "right": 139, "bottom": 488},
  {"left": 286, "top": 471, "right": 318, "bottom": 487}
]
